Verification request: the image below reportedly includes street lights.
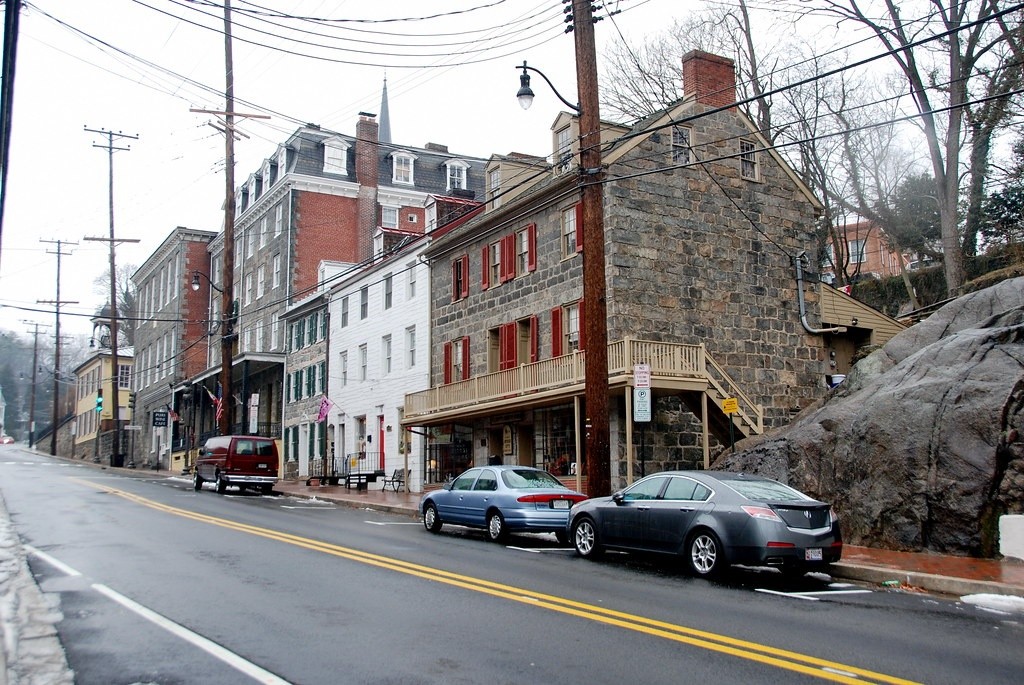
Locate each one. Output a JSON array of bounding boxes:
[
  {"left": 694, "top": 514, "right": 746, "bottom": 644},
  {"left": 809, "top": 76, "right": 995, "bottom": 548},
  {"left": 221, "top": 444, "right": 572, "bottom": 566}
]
[
  {"left": 192, "top": 270, "right": 235, "bottom": 436},
  {"left": 39, "top": 365, "right": 59, "bottom": 456},
  {"left": 20, "top": 372, "right": 36, "bottom": 448},
  {"left": 515, "top": 60, "right": 613, "bottom": 500},
  {"left": 90, "top": 336, "right": 119, "bottom": 467}
]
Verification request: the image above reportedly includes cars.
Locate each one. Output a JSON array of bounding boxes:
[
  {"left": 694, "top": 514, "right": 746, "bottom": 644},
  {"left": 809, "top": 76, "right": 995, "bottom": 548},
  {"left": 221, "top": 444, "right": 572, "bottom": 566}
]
[
  {"left": 567, "top": 471, "right": 843, "bottom": 580},
  {"left": 419, "top": 465, "right": 589, "bottom": 543}
]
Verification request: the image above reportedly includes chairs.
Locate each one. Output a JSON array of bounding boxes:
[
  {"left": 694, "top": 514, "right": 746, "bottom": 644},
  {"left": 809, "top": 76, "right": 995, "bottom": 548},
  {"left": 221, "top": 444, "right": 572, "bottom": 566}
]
[{"left": 381, "top": 469, "right": 411, "bottom": 493}]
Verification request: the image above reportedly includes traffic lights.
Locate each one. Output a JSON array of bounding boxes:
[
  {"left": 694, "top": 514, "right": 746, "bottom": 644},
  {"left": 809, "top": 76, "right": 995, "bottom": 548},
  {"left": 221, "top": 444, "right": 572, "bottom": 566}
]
[
  {"left": 97, "top": 397, "right": 103, "bottom": 412},
  {"left": 128, "top": 392, "right": 136, "bottom": 409}
]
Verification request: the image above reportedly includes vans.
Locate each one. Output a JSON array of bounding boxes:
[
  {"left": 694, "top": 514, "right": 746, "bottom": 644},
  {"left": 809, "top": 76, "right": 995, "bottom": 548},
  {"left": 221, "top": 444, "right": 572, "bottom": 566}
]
[{"left": 193, "top": 435, "right": 279, "bottom": 493}]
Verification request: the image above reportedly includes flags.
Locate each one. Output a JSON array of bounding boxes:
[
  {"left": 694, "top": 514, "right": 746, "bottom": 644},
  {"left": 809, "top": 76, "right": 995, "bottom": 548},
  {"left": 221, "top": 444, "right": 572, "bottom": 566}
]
[
  {"left": 317, "top": 393, "right": 334, "bottom": 424},
  {"left": 207, "top": 385, "right": 226, "bottom": 424},
  {"left": 166, "top": 404, "right": 181, "bottom": 422}
]
